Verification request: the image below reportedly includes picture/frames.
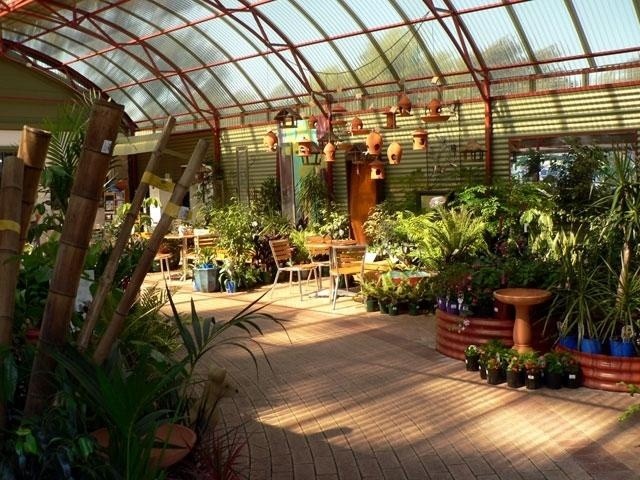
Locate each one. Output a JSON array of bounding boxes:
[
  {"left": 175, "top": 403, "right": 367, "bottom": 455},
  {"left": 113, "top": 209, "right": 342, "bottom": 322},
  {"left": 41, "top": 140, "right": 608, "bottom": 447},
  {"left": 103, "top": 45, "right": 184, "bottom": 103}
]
[{"left": 103, "top": 192, "right": 116, "bottom": 225}]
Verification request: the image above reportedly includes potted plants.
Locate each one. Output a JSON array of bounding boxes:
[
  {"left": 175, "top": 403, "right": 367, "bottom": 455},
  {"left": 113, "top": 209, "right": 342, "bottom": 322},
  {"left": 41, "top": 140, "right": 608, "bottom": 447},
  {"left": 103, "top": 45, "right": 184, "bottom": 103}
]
[
  {"left": 189, "top": 196, "right": 289, "bottom": 292},
  {"left": 540, "top": 142, "right": 640, "bottom": 357},
  {"left": 351, "top": 202, "right": 501, "bottom": 318}
]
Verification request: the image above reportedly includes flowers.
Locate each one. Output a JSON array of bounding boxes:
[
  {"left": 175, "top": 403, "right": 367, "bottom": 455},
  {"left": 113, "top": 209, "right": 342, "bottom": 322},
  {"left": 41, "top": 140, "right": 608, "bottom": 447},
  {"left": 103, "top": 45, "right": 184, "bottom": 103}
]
[{"left": 467, "top": 339, "right": 574, "bottom": 372}]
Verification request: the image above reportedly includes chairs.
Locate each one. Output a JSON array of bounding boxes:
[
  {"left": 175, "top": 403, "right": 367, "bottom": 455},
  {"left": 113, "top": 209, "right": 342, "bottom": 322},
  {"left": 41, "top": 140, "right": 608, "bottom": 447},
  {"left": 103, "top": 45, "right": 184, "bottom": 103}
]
[
  {"left": 145, "top": 232, "right": 218, "bottom": 282},
  {"left": 269, "top": 235, "right": 366, "bottom": 310}
]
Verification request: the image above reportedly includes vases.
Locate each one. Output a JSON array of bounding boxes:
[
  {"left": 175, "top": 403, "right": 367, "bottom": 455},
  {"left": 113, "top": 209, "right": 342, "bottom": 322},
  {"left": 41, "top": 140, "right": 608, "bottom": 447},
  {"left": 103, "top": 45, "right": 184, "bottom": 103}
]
[{"left": 464, "top": 353, "right": 581, "bottom": 390}]
